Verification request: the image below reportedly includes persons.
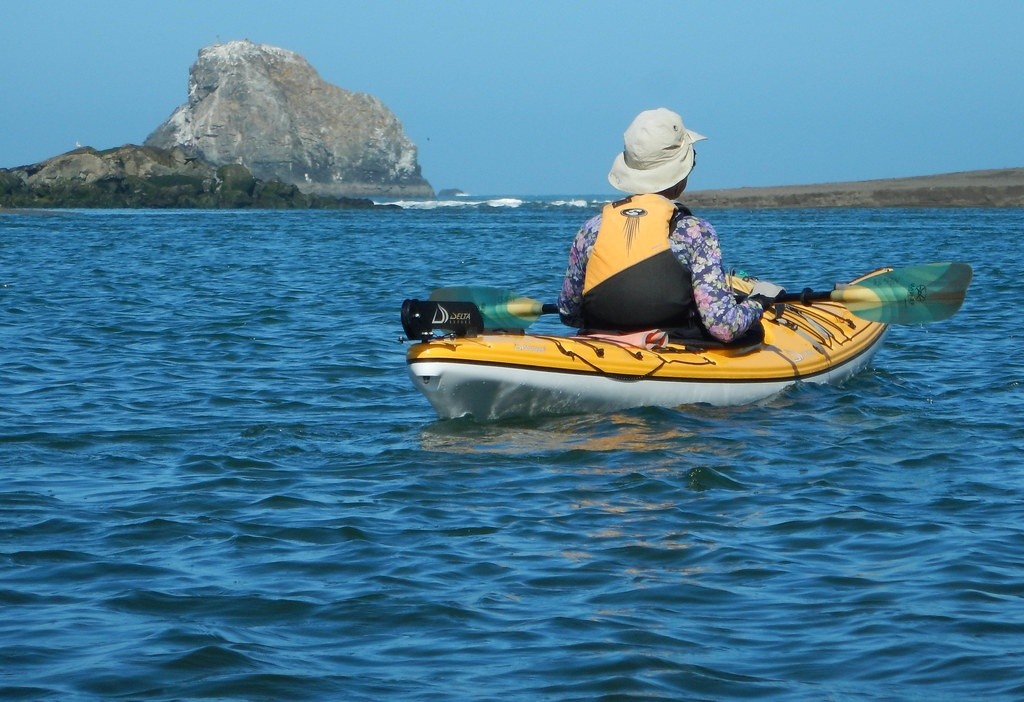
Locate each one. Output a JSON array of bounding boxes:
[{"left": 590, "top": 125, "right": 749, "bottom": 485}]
[{"left": 557, "top": 109, "right": 786, "bottom": 344}]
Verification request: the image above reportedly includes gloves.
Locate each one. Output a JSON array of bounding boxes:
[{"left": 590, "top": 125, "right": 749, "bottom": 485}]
[{"left": 748, "top": 282, "right": 788, "bottom": 309}]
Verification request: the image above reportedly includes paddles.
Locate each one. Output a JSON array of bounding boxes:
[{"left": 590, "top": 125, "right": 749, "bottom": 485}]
[{"left": 427, "top": 260, "right": 974, "bottom": 336}]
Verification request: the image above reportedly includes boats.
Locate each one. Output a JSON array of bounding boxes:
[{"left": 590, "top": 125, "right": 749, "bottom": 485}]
[{"left": 400, "top": 291, "right": 893, "bottom": 422}]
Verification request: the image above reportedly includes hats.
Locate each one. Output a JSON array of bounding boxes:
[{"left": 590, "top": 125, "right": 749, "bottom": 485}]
[{"left": 607, "top": 109, "right": 707, "bottom": 192}]
